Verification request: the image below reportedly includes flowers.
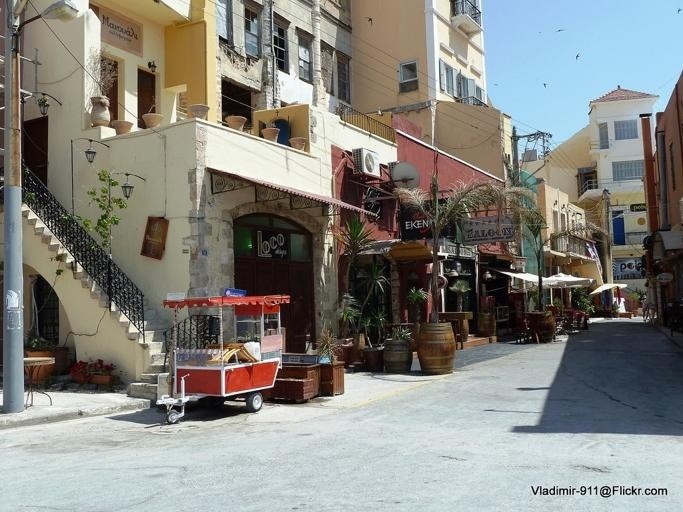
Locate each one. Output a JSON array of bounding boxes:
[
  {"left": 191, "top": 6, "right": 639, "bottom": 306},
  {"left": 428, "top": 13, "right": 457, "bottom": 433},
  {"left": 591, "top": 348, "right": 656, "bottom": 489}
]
[{"left": 68, "top": 358, "right": 118, "bottom": 382}]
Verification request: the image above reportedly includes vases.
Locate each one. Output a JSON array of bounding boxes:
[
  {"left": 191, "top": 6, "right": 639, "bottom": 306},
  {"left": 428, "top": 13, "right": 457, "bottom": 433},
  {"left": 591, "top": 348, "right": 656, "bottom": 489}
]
[
  {"left": 111, "top": 103, "right": 210, "bottom": 135},
  {"left": 223, "top": 114, "right": 307, "bottom": 152},
  {"left": 93, "top": 374, "right": 114, "bottom": 385}
]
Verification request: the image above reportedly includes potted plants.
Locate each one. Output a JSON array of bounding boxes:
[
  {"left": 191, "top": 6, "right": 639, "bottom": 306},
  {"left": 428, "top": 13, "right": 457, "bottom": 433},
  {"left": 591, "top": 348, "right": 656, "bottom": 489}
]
[
  {"left": 86, "top": 47, "right": 122, "bottom": 127},
  {"left": 314, "top": 208, "right": 413, "bottom": 396},
  {"left": 25, "top": 335, "right": 58, "bottom": 385},
  {"left": 392, "top": 176, "right": 599, "bottom": 377}
]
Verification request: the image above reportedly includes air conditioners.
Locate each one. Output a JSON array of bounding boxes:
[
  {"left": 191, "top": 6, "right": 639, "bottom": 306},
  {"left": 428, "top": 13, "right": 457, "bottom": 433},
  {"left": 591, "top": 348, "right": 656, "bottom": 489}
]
[{"left": 351, "top": 147, "right": 382, "bottom": 180}]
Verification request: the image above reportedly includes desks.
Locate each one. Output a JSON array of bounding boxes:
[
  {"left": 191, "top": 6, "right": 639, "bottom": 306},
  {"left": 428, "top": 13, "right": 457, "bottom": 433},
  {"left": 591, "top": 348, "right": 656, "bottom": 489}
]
[{"left": 20, "top": 355, "right": 55, "bottom": 411}]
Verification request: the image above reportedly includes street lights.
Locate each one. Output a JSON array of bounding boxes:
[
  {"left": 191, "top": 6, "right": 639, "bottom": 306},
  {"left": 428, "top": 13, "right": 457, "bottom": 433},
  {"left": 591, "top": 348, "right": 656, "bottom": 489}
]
[{"left": 2, "top": 1, "right": 82, "bottom": 414}]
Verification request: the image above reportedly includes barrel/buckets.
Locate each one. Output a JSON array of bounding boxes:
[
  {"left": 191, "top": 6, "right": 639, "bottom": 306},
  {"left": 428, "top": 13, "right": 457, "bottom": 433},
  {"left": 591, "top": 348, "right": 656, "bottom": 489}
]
[
  {"left": 477, "top": 312, "right": 496, "bottom": 336},
  {"left": 417, "top": 323, "right": 456, "bottom": 375},
  {"left": 532, "top": 313, "right": 555, "bottom": 342}
]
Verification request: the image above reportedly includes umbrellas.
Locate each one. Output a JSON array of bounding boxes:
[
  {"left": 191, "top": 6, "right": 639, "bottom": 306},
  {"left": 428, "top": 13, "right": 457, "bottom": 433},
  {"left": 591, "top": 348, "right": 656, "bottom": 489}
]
[
  {"left": 534, "top": 272, "right": 597, "bottom": 317},
  {"left": 589, "top": 282, "right": 628, "bottom": 297}
]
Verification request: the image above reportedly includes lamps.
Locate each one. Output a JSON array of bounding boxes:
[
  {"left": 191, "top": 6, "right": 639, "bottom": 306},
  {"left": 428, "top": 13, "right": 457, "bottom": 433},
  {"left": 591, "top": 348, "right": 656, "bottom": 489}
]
[
  {"left": 36, "top": 87, "right": 64, "bottom": 118},
  {"left": 83, "top": 137, "right": 146, "bottom": 198},
  {"left": 148, "top": 59, "right": 158, "bottom": 73}
]
